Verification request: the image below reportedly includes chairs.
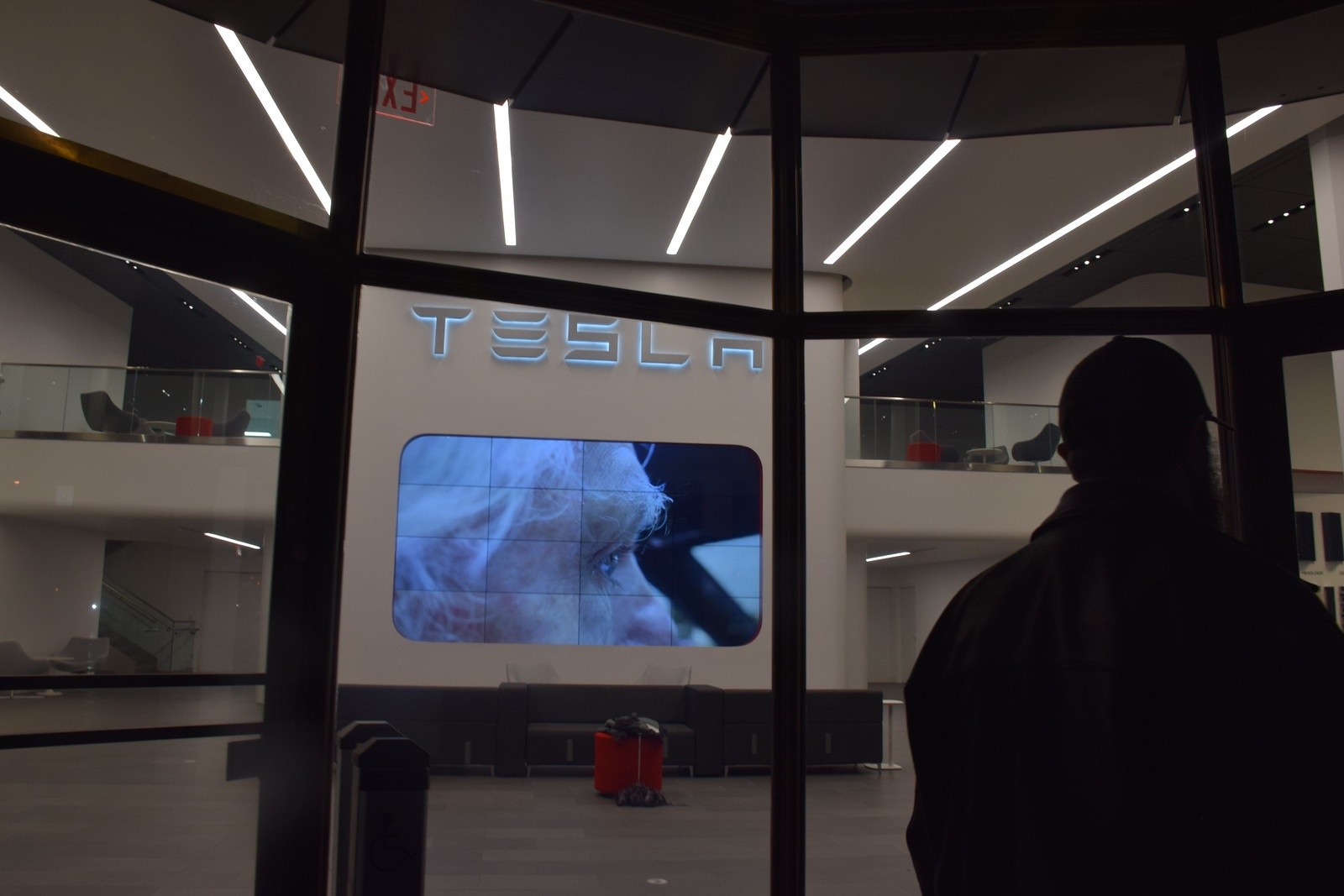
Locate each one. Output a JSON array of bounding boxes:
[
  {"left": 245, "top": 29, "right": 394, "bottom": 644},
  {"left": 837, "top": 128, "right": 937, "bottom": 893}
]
[
  {"left": 76, "top": 389, "right": 141, "bottom": 434},
  {"left": 208, "top": 409, "right": 252, "bottom": 440},
  {"left": 49, "top": 636, "right": 112, "bottom": 677},
  {"left": 0, "top": 639, "right": 58, "bottom": 699},
  {"left": 1011, "top": 423, "right": 1062, "bottom": 474}
]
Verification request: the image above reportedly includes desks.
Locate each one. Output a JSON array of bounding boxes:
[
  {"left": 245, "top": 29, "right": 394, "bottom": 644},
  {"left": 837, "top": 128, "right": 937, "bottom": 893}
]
[
  {"left": 864, "top": 696, "right": 906, "bottom": 770},
  {"left": 147, "top": 415, "right": 210, "bottom": 441},
  {"left": 23, "top": 651, "right": 76, "bottom": 697},
  {"left": 959, "top": 445, "right": 1009, "bottom": 470}
]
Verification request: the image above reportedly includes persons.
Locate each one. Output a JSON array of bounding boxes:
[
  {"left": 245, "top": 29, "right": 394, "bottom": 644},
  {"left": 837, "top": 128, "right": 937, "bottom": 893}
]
[
  {"left": 393, "top": 433, "right": 679, "bottom": 647},
  {"left": 890, "top": 334, "right": 1344, "bottom": 895}
]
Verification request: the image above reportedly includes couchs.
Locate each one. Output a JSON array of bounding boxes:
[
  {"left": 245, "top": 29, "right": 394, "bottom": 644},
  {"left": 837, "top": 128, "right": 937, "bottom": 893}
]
[
  {"left": 321, "top": 679, "right": 887, "bottom": 775},
  {"left": 907, "top": 442, "right": 959, "bottom": 465}
]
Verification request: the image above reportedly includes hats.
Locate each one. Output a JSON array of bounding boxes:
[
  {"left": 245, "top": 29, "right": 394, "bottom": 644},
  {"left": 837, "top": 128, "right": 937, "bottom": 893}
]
[{"left": 1059, "top": 335, "right": 1239, "bottom": 440}]
